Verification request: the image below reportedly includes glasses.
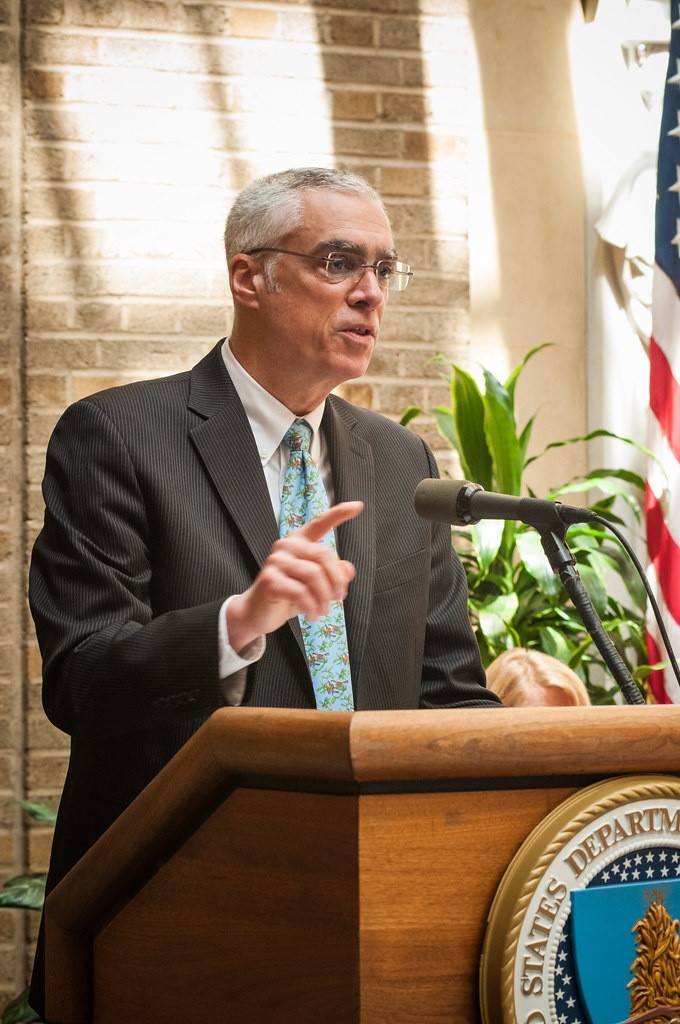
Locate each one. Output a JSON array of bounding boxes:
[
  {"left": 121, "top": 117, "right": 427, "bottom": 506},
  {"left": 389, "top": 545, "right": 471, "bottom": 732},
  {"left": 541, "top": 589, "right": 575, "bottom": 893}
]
[{"left": 246, "top": 247, "right": 413, "bottom": 291}]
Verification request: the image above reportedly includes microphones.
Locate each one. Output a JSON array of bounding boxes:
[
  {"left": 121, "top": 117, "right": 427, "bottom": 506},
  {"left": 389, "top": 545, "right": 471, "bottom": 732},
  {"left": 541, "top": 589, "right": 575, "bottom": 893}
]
[{"left": 414, "top": 478, "right": 597, "bottom": 527}]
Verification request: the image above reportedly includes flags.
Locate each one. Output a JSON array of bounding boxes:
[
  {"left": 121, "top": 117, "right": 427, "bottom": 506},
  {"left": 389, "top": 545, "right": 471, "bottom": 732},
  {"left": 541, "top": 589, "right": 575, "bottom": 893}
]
[{"left": 641, "top": 0, "right": 680, "bottom": 705}]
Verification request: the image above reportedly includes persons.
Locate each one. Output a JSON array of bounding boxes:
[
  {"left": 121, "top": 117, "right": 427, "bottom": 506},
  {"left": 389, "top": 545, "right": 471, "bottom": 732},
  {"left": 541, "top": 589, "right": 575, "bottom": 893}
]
[
  {"left": 483, "top": 646, "right": 593, "bottom": 706},
  {"left": 29, "top": 169, "right": 506, "bottom": 1024}
]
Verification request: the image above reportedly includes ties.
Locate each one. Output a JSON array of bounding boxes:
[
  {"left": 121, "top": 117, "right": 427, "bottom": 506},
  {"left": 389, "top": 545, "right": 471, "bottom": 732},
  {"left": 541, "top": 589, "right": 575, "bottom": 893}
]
[{"left": 282, "top": 420, "right": 355, "bottom": 714}]
